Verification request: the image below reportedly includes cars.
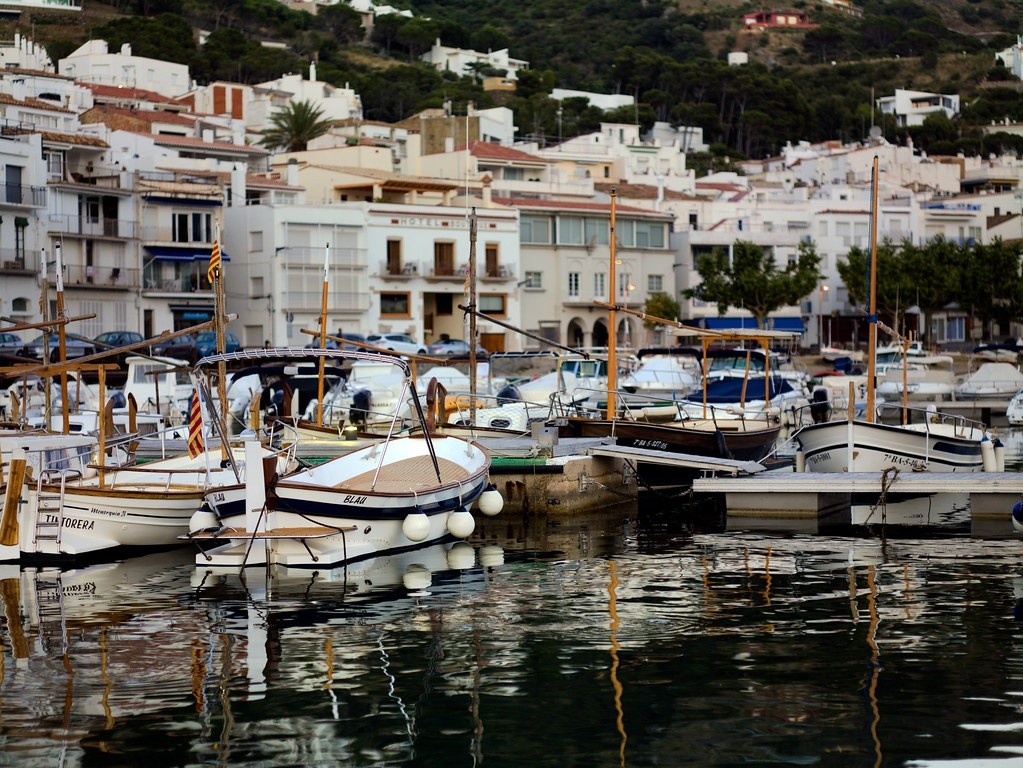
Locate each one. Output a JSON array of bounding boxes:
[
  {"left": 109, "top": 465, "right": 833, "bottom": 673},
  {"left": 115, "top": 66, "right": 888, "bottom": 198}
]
[
  {"left": 305, "top": 333, "right": 367, "bottom": 352},
  {"left": 24, "top": 331, "right": 97, "bottom": 363},
  {"left": 0, "top": 333, "right": 24, "bottom": 358},
  {"left": 428, "top": 339, "right": 489, "bottom": 359},
  {"left": 93, "top": 331, "right": 150, "bottom": 372},
  {"left": 194, "top": 332, "right": 239, "bottom": 357},
  {"left": 145, "top": 332, "right": 199, "bottom": 367},
  {"left": 365, "top": 334, "right": 430, "bottom": 361}
]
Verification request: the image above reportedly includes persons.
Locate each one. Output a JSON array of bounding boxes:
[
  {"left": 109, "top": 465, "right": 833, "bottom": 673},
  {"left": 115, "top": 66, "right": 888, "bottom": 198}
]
[{"left": 264, "top": 327, "right": 485, "bottom": 357}]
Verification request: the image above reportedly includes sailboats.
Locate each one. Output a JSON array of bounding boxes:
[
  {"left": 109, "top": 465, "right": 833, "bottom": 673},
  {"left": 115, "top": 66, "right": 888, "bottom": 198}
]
[
  {"left": 0, "top": 154, "right": 1023, "bottom": 571},
  {"left": 1, "top": 533, "right": 1023, "bottom": 768}
]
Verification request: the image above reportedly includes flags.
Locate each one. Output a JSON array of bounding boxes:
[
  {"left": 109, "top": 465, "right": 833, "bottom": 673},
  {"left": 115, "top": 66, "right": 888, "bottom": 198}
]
[
  {"left": 187, "top": 385, "right": 206, "bottom": 460},
  {"left": 207, "top": 228, "right": 230, "bottom": 284}
]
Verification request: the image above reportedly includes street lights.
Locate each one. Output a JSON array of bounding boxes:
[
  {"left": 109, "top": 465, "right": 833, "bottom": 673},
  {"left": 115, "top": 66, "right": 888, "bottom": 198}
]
[
  {"left": 816, "top": 287, "right": 830, "bottom": 352},
  {"left": 623, "top": 283, "right": 636, "bottom": 348}
]
